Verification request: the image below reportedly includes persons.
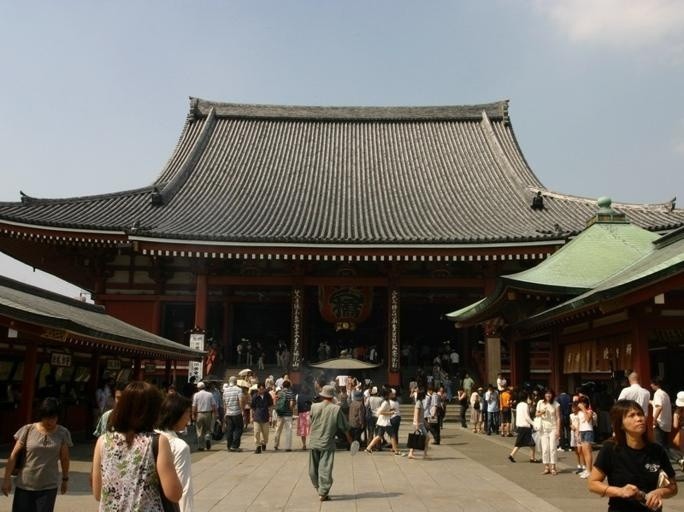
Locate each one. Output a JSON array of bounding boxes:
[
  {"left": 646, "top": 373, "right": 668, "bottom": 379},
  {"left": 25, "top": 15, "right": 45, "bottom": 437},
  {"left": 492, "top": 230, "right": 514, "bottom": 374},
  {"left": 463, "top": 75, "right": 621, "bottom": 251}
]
[
  {"left": 587, "top": 399, "right": 678, "bottom": 512},
  {"left": 307, "top": 385, "right": 348, "bottom": 502},
  {"left": 2, "top": 397, "right": 74, "bottom": 512},
  {"left": 94, "top": 338, "right": 684, "bottom": 478},
  {"left": 153, "top": 392, "right": 193, "bottom": 512},
  {"left": 92, "top": 380, "right": 183, "bottom": 512}
]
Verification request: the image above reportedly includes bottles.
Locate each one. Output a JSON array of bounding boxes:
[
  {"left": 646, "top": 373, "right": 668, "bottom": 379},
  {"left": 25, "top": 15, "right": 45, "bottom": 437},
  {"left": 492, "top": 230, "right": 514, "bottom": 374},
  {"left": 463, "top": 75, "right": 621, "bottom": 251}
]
[{"left": 632, "top": 488, "right": 662, "bottom": 512}]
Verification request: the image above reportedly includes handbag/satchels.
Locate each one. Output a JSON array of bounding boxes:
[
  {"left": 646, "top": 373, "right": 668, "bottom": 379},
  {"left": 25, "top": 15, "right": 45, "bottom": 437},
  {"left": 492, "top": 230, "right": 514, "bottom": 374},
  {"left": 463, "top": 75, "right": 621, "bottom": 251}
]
[
  {"left": 11, "top": 447, "right": 26, "bottom": 475},
  {"left": 474, "top": 400, "right": 480, "bottom": 409},
  {"left": 436, "top": 407, "right": 445, "bottom": 418},
  {"left": 158, "top": 480, "right": 180, "bottom": 512},
  {"left": 213, "top": 419, "right": 223, "bottom": 441},
  {"left": 407, "top": 430, "right": 425, "bottom": 450},
  {"left": 533, "top": 417, "right": 541, "bottom": 430}
]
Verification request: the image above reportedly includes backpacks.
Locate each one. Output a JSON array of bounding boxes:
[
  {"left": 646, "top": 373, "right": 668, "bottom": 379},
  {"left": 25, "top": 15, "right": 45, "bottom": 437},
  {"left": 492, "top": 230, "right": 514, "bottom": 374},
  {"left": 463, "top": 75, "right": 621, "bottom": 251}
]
[{"left": 276, "top": 391, "right": 288, "bottom": 415}]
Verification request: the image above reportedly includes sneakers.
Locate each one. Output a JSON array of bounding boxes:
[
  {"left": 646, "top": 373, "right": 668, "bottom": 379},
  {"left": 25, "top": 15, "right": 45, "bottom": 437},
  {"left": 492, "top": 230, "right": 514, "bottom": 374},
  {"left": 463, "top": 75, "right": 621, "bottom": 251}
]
[
  {"left": 319, "top": 495, "right": 330, "bottom": 500},
  {"left": 574, "top": 467, "right": 591, "bottom": 478},
  {"left": 228, "top": 447, "right": 242, "bottom": 452},
  {"left": 394, "top": 451, "right": 431, "bottom": 458},
  {"left": 509, "top": 455, "right": 515, "bottom": 462},
  {"left": 557, "top": 446, "right": 572, "bottom": 451},
  {"left": 530, "top": 459, "right": 540, "bottom": 463},
  {"left": 274, "top": 446, "right": 291, "bottom": 451},
  {"left": 365, "top": 449, "right": 374, "bottom": 455},
  {"left": 432, "top": 441, "right": 440, "bottom": 444},
  {"left": 544, "top": 467, "right": 557, "bottom": 475},
  {"left": 255, "top": 444, "right": 266, "bottom": 453},
  {"left": 199, "top": 439, "right": 210, "bottom": 450},
  {"left": 473, "top": 427, "right": 513, "bottom": 437}
]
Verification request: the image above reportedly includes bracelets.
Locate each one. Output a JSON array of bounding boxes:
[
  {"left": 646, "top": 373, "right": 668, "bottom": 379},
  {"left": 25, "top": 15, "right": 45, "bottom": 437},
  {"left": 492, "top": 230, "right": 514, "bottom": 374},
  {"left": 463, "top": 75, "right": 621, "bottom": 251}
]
[
  {"left": 601, "top": 486, "right": 609, "bottom": 497},
  {"left": 62, "top": 477, "right": 69, "bottom": 481}
]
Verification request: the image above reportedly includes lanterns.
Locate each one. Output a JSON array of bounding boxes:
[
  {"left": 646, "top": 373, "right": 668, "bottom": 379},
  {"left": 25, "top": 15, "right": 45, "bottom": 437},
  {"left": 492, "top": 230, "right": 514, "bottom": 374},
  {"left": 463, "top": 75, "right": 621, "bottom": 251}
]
[{"left": 318, "top": 263, "right": 374, "bottom": 335}]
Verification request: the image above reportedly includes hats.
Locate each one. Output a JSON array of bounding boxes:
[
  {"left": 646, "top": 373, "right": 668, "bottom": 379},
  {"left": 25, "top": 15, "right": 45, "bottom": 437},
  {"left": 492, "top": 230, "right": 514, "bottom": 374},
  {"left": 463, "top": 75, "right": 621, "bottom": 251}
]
[
  {"left": 676, "top": 391, "right": 684, "bottom": 407},
  {"left": 319, "top": 385, "right": 334, "bottom": 398},
  {"left": 353, "top": 390, "right": 362, "bottom": 401},
  {"left": 197, "top": 382, "right": 205, "bottom": 389}
]
